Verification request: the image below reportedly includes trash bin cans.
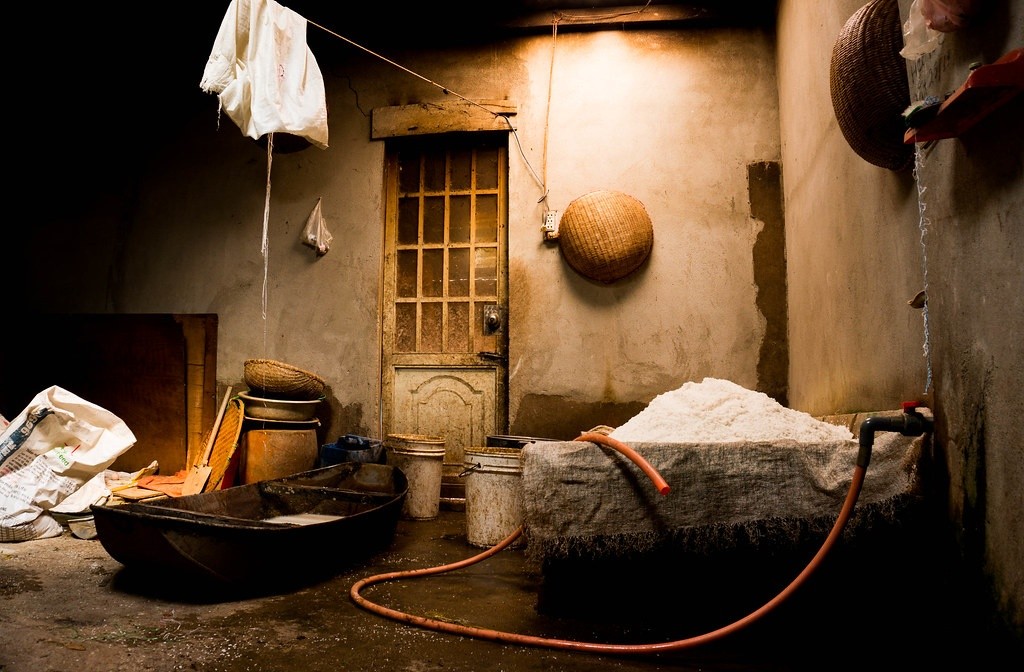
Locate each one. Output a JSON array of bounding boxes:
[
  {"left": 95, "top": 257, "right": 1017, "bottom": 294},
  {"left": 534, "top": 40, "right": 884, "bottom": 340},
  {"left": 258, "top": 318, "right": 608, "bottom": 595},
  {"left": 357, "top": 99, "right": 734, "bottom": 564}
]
[{"left": 319, "top": 434, "right": 382, "bottom": 467}]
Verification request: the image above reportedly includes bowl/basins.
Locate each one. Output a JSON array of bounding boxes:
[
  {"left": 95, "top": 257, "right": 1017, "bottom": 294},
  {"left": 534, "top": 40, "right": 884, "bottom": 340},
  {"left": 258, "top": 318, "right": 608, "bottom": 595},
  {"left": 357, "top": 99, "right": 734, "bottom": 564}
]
[{"left": 239, "top": 390, "right": 321, "bottom": 421}]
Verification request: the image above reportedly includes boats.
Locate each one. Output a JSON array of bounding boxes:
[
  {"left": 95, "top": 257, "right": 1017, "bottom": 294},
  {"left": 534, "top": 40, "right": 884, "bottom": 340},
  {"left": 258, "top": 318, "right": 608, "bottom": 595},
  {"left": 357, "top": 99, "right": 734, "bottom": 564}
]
[{"left": 88, "top": 460, "right": 411, "bottom": 592}]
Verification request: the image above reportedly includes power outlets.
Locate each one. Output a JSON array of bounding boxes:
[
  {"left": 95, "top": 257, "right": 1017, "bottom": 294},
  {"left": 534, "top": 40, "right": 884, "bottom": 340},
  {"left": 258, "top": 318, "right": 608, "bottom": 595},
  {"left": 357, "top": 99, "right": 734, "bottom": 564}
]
[{"left": 545, "top": 211, "right": 556, "bottom": 232}]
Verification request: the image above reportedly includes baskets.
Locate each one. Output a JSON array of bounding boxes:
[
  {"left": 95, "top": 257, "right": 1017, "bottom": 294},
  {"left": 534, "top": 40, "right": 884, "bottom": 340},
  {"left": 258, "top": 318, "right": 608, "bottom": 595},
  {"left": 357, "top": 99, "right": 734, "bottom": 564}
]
[
  {"left": 558, "top": 189, "right": 653, "bottom": 285},
  {"left": 830, "top": 0, "right": 915, "bottom": 173},
  {"left": 243, "top": 359, "right": 325, "bottom": 401}
]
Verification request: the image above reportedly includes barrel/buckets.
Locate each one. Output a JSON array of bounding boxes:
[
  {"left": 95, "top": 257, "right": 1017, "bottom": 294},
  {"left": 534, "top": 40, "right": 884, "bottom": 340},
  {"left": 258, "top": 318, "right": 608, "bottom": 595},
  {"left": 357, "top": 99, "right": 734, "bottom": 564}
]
[
  {"left": 462, "top": 446, "right": 526, "bottom": 550},
  {"left": 485, "top": 434, "right": 558, "bottom": 448},
  {"left": 384, "top": 432, "right": 446, "bottom": 519}
]
